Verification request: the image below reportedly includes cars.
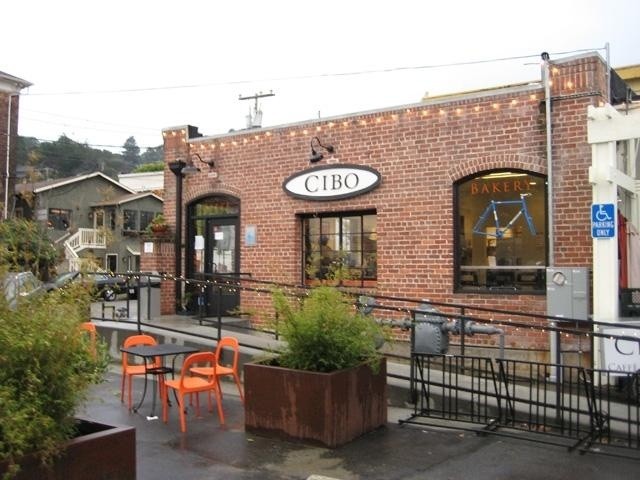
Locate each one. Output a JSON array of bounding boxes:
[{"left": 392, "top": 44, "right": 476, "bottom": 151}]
[
  {"left": 44, "top": 272, "right": 127, "bottom": 301},
  {"left": 127, "top": 269, "right": 162, "bottom": 300}
]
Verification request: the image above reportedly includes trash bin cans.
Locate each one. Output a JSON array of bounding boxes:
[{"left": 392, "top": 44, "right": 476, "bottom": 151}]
[{"left": 140, "top": 270, "right": 161, "bottom": 320}]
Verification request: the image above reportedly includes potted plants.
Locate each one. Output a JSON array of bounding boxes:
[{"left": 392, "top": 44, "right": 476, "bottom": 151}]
[
  {"left": 145, "top": 214, "right": 167, "bottom": 239},
  {"left": 228, "top": 244, "right": 420, "bottom": 450},
  {"left": 1, "top": 148, "right": 136, "bottom": 480}
]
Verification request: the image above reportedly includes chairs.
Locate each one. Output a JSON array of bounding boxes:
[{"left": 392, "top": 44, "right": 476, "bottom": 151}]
[
  {"left": 121, "top": 335, "right": 245, "bottom": 433},
  {"left": 73, "top": 322, "right": 96, "bottom": 360}
]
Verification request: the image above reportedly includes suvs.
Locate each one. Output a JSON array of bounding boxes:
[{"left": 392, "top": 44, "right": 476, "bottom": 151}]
[{"left": 1, "top": 271, "right": 45, "bottom": 312}]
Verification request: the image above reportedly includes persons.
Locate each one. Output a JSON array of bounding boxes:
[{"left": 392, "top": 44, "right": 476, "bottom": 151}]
[{"left": 314, "top": 235, "right": 332, "bottom": 259}]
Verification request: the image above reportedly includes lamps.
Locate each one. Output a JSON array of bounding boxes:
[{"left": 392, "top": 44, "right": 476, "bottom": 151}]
[
  {"left": 181, "top": 153, "right": 215, "bottom": 174},
  {"left": 310, "top": 136, "right": 334, "bottom": 162}
]
